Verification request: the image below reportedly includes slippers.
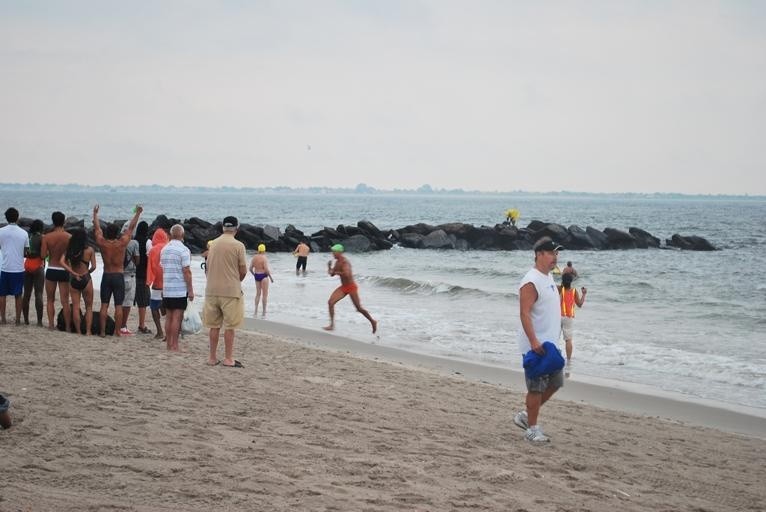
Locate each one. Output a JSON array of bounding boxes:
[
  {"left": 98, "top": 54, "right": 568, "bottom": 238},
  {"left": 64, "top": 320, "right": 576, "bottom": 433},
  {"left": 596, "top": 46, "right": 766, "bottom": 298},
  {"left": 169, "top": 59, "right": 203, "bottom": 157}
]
[
  {"left": 222, "top": 360, "right": 243, "bottom": 367},
  {"left": 206, "top": 358, "right": 220, "bottom": 366}
]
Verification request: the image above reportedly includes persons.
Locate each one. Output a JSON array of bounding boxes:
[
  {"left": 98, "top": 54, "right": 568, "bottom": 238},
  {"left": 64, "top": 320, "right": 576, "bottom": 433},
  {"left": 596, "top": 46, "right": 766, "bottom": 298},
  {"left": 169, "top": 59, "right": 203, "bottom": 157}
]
[
  {"left": 562, "top": 261, "right": 577, "bottom": 279},
  {"left": 202, "top": 216, "right": 247, "bottom": 369},
  {"left": 0, "top": 393, "right": 12, "bottom": 429},
  {"left": 556, "top": 273, "right": 587, "bottom": 365},
  {"left": 322, "top": 244, "right": 377, "bottom": 334},
  {"left": 0, "top": 204, "right": 194, "bottom": 351},
  {"left": 249, "top": 244, "right": 273, "bottom": 318},
  {"left": 293, "top": 240, "right": 310, "bottom": 273},
  {"left": 504, "top": 208, "right": 520, "bottom": 226},
  {"left": 514, "top": 236, "right": 565, "bottom": 443}
]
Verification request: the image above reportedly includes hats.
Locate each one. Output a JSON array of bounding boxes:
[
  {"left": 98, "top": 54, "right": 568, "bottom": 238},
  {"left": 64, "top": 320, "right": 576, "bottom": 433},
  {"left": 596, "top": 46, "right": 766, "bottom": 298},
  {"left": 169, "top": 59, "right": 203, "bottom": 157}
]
[
  {"left": 330, "top": 244, "right": 344, "bottom": 253},
  {"left": 223, "top": 216, "right": 239, "bottom": 228},
  {"left": 533, "top": 236, "right": 564, "bottom": 252},
  {"left": 208, "top": 240, "right": 213, "bottom": 246},
  {"left": 258, "top": 244, "right": 265, "bottom": 252}
]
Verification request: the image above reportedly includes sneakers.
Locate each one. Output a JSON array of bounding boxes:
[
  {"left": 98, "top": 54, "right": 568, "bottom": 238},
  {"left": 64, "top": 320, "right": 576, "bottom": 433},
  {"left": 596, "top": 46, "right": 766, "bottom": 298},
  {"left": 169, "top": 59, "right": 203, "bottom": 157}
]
[
  {"left": 513, "top": 411, "right": 530, "bottom": 429},
  {"left": 114, "top": 324, "right": 138, "bottom": 337},
  {"left": 523, "top": 429, "right": 550, "bottom": 447},
  {"left": 138, "top": 325, "right": 151, "bottom": 333}
]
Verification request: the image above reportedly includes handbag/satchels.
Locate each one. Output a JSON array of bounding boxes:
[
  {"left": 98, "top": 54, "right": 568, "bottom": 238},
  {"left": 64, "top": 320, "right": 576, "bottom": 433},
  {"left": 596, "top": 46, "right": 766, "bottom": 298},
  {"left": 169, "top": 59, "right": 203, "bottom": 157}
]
[
  {"left": 57, "top": 302, "right": 85, "bottom": 334},
  {"left": 80, "top": 312, "right": 116, "bottom": 336},
  {"left": 522, "top": 341, "right": 565, "bottom": 379}
]
